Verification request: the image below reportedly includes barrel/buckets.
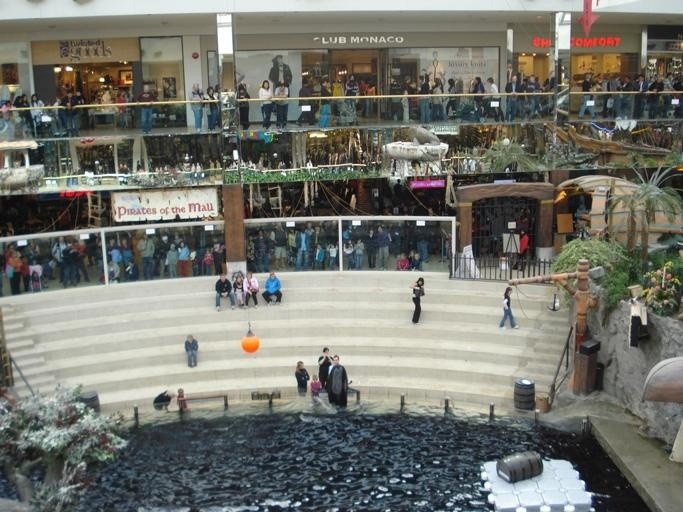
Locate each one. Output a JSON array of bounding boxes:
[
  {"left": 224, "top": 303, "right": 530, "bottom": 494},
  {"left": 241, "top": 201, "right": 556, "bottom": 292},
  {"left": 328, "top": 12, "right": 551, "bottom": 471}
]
[
  {"left": 514, "top": 380, "right": 535, "bottom": 410},
  {"left": 497, "top": 451, "right": 543, "bottom": 484},
  {"left": 78, "top": 392, "right": 100, "bottom": 413}
]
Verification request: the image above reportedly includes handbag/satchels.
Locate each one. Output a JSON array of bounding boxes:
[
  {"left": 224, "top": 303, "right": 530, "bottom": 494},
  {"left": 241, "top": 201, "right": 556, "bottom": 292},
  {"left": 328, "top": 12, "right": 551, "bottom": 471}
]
[{"left": 5, "top": 265, "right": 14, "bottom": 278}]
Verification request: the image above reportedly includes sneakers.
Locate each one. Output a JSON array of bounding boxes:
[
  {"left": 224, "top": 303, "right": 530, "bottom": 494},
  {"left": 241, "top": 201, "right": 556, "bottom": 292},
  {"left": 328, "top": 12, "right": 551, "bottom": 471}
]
[
  {"left": 216, "top": 302, "right": 282, "bottom": 311},
  {"left": 499, "top": 325, "right": 520, "bottom": 330}
]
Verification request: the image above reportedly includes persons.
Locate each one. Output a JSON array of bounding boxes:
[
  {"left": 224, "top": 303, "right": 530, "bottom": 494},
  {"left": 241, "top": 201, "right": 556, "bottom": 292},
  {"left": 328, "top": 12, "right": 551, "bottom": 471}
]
[
  {"left": 230, "top": 277, "right": 245, "bottom": 309},
  {"left": 310, "top": 374, "right": 323, "bottom": 399},
  {"left": 176, "top": 388, "right": 187, "bottom": 412},
  {"left": 498, "top": 288, "right": 518, "bottom": 330},
  {"left": 317, "top": 346, "right": 334, "bottom": 390},
  {"left": 267, "top": 55, "right": 292, "bottom": 90},
  {"left": 0, "top": 72, "right": 683, "bottom": 288},
  {"left": 214, "top": 273, "right": 235, "bottom": 313},
  {"left": 182, "top": 334, "right": 198, "bottom": 369},
  {"left": 424, "top": 51, "right": 447, "bottom": 88},
  {"left": 260, "top": 272, "right": 282, "bottom": 306},
  {"left": 325, "top": 355, "right": 349, "bottom": 409},
  {"left": 242, "top": 271, "right": 259, "bottom": 309},
  {"left": 294, "top": 361, "right": 310, "bottom": 396},
  {"left": 409, "top": 277, "right": 425, "bottom": 323}
]
